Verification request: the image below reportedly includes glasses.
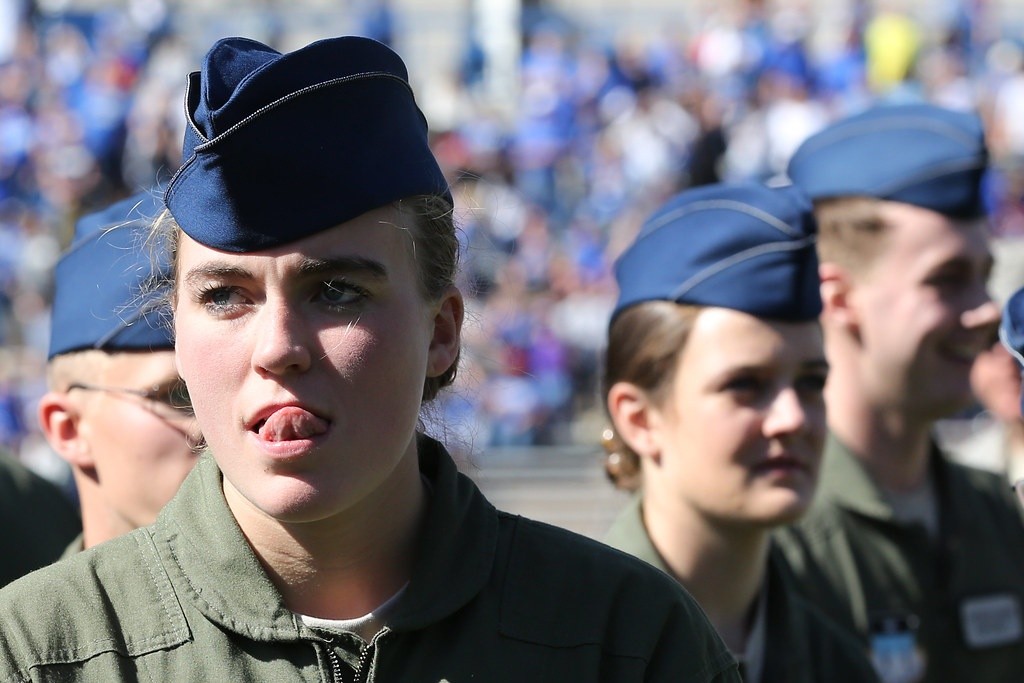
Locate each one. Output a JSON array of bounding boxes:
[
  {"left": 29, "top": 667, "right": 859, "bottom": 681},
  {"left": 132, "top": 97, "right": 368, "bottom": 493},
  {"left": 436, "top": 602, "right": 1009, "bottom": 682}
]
[{"left": 65, "top": 384, "right": 192, "bottom": 412}]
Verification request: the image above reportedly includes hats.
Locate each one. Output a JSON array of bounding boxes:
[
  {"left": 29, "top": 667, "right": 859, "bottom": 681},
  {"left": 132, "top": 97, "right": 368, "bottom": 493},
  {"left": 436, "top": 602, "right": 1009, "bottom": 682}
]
[
  {"left": 998, "top": 285, "right": 1024, "bottom": 366},
  {"left": 47, "top": 181, "right": 174, "bottom": 356},
  {"left": 787, "top": 99, "right": 989, "bottom": 221},
  {"left": 165, "top": 36, "right": 453, "bottom": 253},
  {"left": 607, "top": 182, "right": 824, "bottom": 322}
]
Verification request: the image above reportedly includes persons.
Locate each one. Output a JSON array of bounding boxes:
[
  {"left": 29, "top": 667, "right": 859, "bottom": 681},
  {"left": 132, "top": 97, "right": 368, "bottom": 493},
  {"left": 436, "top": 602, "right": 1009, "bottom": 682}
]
[
  {"left": 38, "top": 179, "right": 208, "bottom": 568},
  {"left": 0, "top": 0, "right": 1024, "bottom": 495},
  {"left": 0, "top": 33, "right": 741, "bottom": 683},
  {"left": 603, "top": 186, "right": 877, "bottom": 683},
  {"left": 784, "top": 104, "right": 1024, "bottom": 683}
]
